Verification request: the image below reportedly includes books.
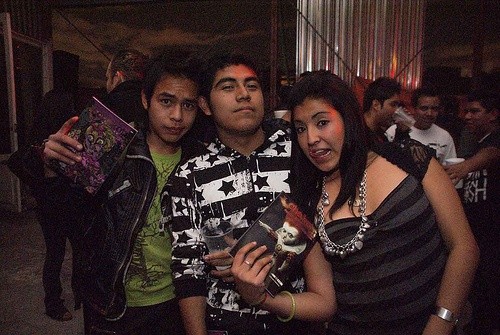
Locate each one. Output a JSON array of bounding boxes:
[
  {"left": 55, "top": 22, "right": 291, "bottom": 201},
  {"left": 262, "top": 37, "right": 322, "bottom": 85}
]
[{"left": 48, "top": 97, "right": 139, "bottom": 196}]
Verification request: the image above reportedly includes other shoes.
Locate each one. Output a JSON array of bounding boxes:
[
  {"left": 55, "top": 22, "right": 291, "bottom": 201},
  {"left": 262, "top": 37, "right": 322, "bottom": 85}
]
[{"left": 45, "top": 303, "right": 74, "bottom": 322}]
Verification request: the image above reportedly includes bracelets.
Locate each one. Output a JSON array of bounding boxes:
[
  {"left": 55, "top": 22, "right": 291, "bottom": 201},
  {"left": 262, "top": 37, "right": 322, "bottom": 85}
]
[
  {"left": 276, "top": 291, "right": 295, "bottom": 323},
  {"left": 431, "top": 306, "right": 459, "bottom": 327}
]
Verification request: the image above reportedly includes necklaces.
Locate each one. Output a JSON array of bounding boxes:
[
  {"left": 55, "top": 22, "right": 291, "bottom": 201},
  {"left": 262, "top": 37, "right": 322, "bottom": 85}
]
[{"left": 316, "top": 169, "right": 371, "bottom": 256}]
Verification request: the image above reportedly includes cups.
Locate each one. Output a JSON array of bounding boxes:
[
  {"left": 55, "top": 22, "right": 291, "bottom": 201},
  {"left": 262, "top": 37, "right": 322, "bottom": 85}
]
[
  {"left": 446, "top": 158, "right": 465, "bottom": 188},
  {"left": 392, "top": 107, "right": 416, "bottom": 127},
  {"left": 200, "top": 222, "right": 234, "bottom": 270},
  {"left": 274, "top": 110, "right": 288, "bottom": 118}
]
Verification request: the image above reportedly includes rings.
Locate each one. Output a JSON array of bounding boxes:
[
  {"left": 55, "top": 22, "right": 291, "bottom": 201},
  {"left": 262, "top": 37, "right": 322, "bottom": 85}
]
[{"left": 243, "top": 261, "right": 252, "bottom": 266}]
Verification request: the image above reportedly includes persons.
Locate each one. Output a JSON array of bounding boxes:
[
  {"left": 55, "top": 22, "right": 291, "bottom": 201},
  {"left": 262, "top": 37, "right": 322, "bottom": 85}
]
[
  {"left": 8, "top": 57, "right": 207, "bottom": 335},
  {"left": 231, "top": 69, "right": 500, "bottom": 335},
  {"left": 160, "top": 55, "right": 292, "bottom": 335},
  {"left": 37, "top": 50, "right": 148, "bottom": 321}
]
